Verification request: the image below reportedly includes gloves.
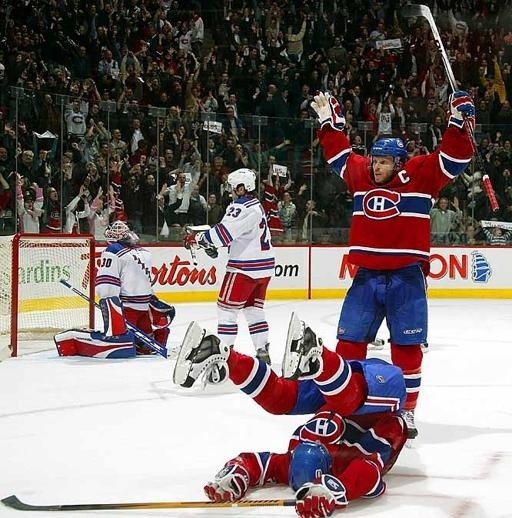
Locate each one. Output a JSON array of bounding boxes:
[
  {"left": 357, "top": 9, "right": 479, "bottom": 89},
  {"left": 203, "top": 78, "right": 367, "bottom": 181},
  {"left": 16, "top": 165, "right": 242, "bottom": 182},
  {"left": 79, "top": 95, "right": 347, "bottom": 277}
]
[
  {"left": 295, "top": 473, "right": 349, "bottom": 518},
  {"left": 310, "top": 91, "right": 347, "bottom": 132},
  {"left": 447, "top": 91, "right": 476, "bottom": 131},
  {"left": 184, "top": 230, "right": 208, "bottom": 249},
  {"left": 203, "top": 452, "right": 262, "bottom": 503}
]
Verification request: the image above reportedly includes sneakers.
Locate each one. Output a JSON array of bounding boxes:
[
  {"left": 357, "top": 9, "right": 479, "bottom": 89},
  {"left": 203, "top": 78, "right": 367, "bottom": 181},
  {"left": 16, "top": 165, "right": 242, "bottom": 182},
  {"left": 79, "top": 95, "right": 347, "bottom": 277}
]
[
  {"left": 287, "top": 327, "right": 323, "bottom": 382},
  {"left": 180, "top": 335, "right": 231, "bottom": 389},
  {"left": 401, "top": 410, "right": 418, "bottom": 436}
]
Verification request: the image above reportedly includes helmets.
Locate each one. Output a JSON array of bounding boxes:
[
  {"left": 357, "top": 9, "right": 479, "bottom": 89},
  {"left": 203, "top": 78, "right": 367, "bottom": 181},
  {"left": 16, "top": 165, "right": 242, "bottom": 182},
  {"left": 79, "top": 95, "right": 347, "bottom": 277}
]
[
  {"left": 289, "top": 441, "right": 333, "bottom": 492},
  {"left": 371, "top": 138, "right": 408, "bottom": 166},
  {"left": 225, "top": 168, "right": 257, "bottom": 193},
  {"left": 104, "top": 220, "right": 140, "bottom": 246}
]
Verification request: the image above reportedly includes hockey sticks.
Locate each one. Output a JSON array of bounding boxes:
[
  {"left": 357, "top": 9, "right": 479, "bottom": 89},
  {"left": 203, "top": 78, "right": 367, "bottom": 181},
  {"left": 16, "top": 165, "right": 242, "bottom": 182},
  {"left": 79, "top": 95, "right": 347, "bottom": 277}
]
[
  {"left": 400, "top": 4, "right": 500, "bottom": 213},
  {"left": 1, "top": 495, "right": 296, "bottom": 511},
  {"left": 60, "top": 278, "right": 182, "bottom": 359},
  {"left": 186, "top": 225, "right": 211, "bottom": 265}
]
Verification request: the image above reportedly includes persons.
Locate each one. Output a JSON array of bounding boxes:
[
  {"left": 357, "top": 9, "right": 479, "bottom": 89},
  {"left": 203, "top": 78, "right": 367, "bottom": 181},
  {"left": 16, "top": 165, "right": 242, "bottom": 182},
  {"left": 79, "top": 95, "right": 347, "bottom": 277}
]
[
  {"left": 306, "top": 86, "right": 481, "bottom": 441},
  {"left": 177, "top": 319, "right": 408, "bottom": 518},
  {"left": 184, "top": 166, "right": 277, "bottom": 368},
  {"left": 1, "top": 1, "right": 511, "bottom": 249},
  {"left": 53, "top": 218, "right": 177, "bottom": 361}
]
